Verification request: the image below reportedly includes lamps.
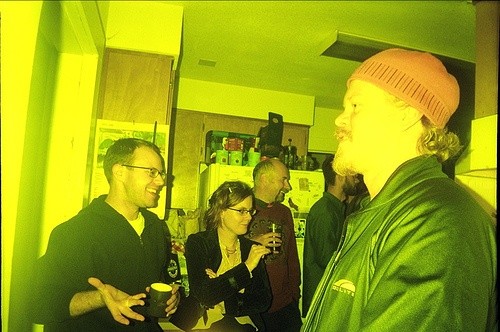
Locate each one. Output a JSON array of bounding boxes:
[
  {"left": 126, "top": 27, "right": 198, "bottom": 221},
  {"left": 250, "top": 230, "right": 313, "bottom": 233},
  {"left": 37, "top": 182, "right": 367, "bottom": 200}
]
[{"left": 318, "top": 30, "right": 476, "bottom": 81}]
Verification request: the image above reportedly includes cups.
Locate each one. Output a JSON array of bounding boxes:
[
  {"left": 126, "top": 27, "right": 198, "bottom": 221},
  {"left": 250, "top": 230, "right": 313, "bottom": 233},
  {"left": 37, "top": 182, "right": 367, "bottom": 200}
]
[
  {"left": 267, "top": 223, "right": 283, "bottom": 254},
  {"left": 215, "top": 150, "right": 228, "bottom": 165},
  {"left": 146, "top": 282, "right": 173, "bottom": 318},
  {"left": 228, "top": 150, "right": 243, "bottom": 166},
  {"left": 247, "top": 152, "right": 261, "bottom": 167}
]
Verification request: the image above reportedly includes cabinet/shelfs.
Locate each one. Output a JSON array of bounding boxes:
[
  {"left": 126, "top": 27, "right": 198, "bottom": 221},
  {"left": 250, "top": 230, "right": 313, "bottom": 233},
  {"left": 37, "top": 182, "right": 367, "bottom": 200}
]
[{"left": 97, "top": 48, "right": 176, "bottom": 129}]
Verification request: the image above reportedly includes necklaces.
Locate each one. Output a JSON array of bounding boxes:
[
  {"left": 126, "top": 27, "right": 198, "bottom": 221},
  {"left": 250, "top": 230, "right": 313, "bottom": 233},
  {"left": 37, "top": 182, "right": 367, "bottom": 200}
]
[{"left": 227, "top": 249, "right": 236, "bottom": 254}]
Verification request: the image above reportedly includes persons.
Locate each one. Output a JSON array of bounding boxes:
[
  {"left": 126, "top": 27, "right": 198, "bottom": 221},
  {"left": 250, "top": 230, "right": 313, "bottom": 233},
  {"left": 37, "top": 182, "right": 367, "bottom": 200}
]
[
  {"left": 302, "top": 154, "right": 360, "bottom": 319},
  {"left": 186, "top": 180, "right": 273, "bottom": 332},
  {"left": 21, "top": 138, "right": 199, "bottom": 332},
  {"left": 301, "top": 48, "right": 498, "bottom": 332},
  {"left": 243, "top": 159, "right": 303, "bottom": 332}
]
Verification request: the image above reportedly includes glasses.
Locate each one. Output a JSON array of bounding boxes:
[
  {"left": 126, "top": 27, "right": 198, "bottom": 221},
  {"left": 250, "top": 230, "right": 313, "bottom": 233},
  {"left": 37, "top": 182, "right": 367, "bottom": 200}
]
[
  {"left": 228, "top": 207, "right": 256, "bottom": 216},
  {"left": 122, "top": 165, "right": 166, "bottom": 180}
]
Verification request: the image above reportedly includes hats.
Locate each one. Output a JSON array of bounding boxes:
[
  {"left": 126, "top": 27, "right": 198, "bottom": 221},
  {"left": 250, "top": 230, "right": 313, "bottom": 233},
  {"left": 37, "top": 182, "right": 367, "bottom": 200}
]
[{"left": 346, "top": 48, "right": 460, "bottom": 130}]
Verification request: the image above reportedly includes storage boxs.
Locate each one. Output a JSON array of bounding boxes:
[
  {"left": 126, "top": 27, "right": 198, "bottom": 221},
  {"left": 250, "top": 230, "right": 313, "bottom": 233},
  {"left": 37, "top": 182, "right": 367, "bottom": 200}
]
[{"left": 204, "top": 130, "right": 257, "bottom": 165}]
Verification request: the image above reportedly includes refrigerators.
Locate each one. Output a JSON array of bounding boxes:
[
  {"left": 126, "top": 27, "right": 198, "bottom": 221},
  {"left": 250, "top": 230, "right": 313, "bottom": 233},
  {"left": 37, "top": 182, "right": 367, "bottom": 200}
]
[{"left": 199, "top": 163, "right": 325, "bottom": 317}]
